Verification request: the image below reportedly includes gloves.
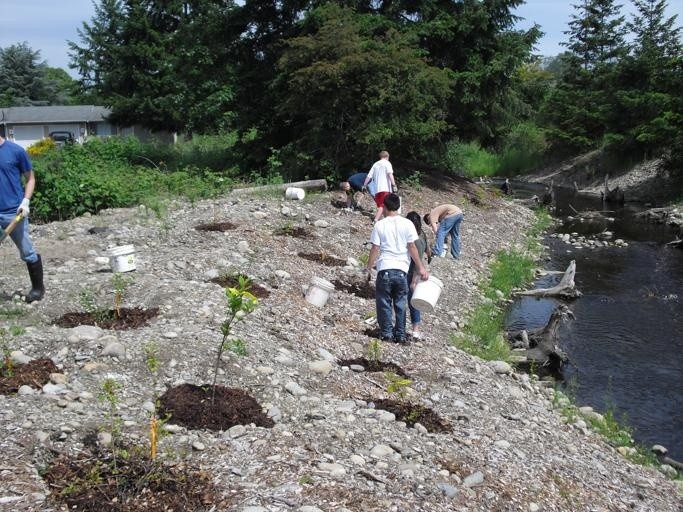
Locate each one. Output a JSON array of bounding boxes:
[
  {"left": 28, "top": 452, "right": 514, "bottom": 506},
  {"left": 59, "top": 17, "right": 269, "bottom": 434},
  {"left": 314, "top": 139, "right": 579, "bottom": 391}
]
[{"left": 17, "top": 198, "right": 30, "bottom": 217}]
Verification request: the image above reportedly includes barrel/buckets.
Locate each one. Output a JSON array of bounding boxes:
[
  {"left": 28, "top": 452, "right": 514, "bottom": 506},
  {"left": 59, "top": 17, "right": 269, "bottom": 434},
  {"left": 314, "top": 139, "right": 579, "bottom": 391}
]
[
  {"left": 106, "top": 244, "right": 135, "bottom": 274},
  {"left": 411, "top": 277, "right": 444, "bottom": 312},
  {"left": 305, "top": 276, "right": 336, "bottom": 307},
  {"left": 285, "top": 187, "right": 305, "bottom": 200}
]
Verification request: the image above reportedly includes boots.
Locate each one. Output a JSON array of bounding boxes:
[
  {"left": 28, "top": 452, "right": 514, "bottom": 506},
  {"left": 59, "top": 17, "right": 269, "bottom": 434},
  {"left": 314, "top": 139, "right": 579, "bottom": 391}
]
[{"left": 26, "top": 254, "right": 44, "bottom": 302}]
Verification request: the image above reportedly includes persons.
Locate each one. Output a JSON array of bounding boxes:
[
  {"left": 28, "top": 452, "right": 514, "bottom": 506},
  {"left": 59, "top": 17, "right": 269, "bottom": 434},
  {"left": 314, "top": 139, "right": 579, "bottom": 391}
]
[
  {"left": 363, "top": 152, "right": 397, "bottom": 223},
  {"left": 366, "top": 194, "right": 428, "bottom": 342},
  {"left": 340, "top": 173, "right": 376, "bottom": 208},
  {"left": 406, "top": 211, "right": 431, "bottom": 338},
  {"left": 423, "top": 203, "right": 464, "bottom": 258},
  {"left": 0, "top": 126, "right": 45, "bottom": 303}
]
